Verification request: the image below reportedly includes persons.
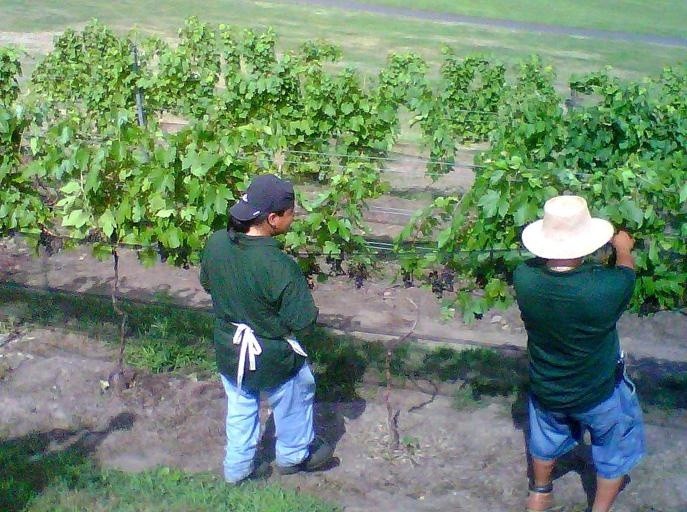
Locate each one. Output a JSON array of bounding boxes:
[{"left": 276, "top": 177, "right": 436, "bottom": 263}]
[
  {"left": 508, "top": 192, "right": 646, "bottom": 512},
  {"left": 194, "top": 171, "right": 336, "bottom": 484}
]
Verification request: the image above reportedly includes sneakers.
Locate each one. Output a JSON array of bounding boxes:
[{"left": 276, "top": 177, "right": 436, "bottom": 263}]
[
  {"left": 276, "top": 441, "right": 333, "bottom": 475},
  {"left": 520, "top": 477, "right": 553, "bottom": 512},
  {"left": 225, "top": 459, "right": 269, "bottom": 488}
]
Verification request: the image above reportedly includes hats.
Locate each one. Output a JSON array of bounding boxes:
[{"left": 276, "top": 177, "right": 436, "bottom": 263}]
[
  {"left": 522, "top": 196, "right": 615, "bottom": 259},
  {"left": 228, "top": 174, "right": 294, "bottom": 220}
]
